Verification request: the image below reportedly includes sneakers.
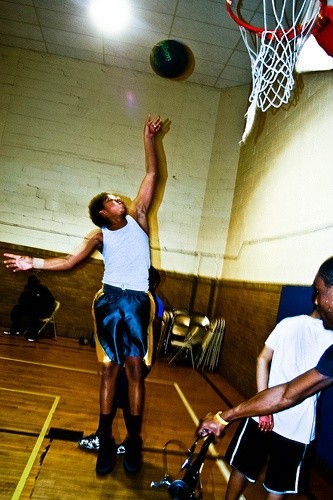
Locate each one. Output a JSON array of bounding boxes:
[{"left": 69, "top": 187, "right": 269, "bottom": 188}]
[
  {"left": 79, "top": 433, "right": 100, "bottom": 452},
  {"left": 124, "top": 438, "right": 142, "bottom": 478},
  {"left": 95, "top": 438, "right": 116, "bottom": 475},
  {"left": 116, "top": 443, "right": 125, "bottom": 454}
]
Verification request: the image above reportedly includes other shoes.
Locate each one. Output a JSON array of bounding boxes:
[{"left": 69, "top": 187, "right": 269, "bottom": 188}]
[
  {"left": 27, "top": 338, "right": 36, "bottom": 342},
  {"left": 3, "top": 330, "right": 19, "bottom": 336}
]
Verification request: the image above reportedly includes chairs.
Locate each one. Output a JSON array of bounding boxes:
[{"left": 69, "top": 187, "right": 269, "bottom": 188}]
[
  {"left": 154, "top": 307, "right": 225, "bottom": 372},
  {"left": 24, "top": 300, "right": 60, "bottom": 342}
]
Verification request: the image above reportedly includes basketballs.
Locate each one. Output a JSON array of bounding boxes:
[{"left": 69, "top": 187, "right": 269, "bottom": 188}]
[{"left": 150, "top": 40, "right": 190, "bottom": 79}]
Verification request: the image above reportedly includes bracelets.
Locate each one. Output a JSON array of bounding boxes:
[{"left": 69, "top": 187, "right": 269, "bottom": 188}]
[{"left": 216, "top": 411, "right": 230, "bottom": 426}]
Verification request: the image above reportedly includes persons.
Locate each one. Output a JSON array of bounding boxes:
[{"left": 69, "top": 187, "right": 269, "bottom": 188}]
[
  {"left": 224, "top": 293, "right": 333, "bottom": 500},
  {"left": 4, "top": 115, "right": 161, "bottom": 474},
  {"left": 199, "top": 256, "right": 333, "bottom": 500}
]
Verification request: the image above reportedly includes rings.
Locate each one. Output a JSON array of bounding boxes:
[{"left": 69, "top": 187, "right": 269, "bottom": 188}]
[{"left": 154, "top": 125, "right": 157, "bottom": 128}]
